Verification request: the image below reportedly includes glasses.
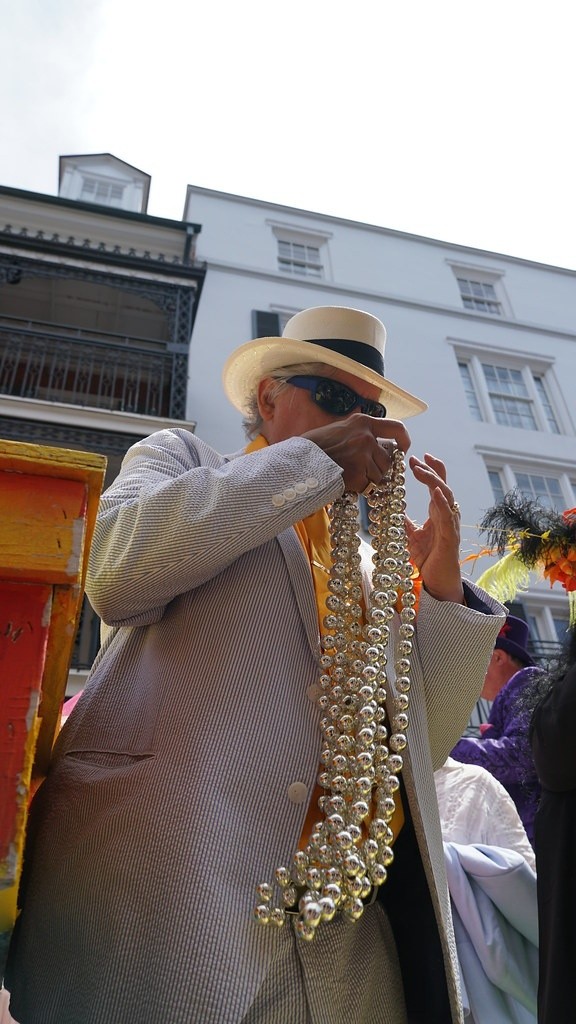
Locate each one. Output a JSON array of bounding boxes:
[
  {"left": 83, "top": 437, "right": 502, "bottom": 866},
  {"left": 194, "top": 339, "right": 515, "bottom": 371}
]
[{"left": 286, "top": 375, "right": 387, "bottom": 418}]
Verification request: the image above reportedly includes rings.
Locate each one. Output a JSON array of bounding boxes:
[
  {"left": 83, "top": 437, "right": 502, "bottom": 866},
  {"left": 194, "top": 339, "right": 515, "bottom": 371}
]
[
  {"left": 362, "top": 482, "right": 376, "bottom": 498},
  {"left": 451, "top": 502, "right": 459, "bottom": 513}
]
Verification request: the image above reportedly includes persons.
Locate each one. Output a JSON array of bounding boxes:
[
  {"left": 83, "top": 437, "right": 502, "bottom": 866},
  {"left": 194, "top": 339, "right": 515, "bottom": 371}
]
[
  {"left": 9, "top": 307, "right": 508, "bottom": 1024},
  {"left": 431, "top": 601, "right": 576, "bottom": 1024}
]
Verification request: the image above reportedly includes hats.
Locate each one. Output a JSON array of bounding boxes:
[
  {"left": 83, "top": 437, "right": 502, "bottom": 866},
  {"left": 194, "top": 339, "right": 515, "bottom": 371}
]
[
  {"left": 493, "top": 614, "right": 536, "bottom": 667},
  {"left": 222, "top": 305, "right": 429, "bottom": 421}
]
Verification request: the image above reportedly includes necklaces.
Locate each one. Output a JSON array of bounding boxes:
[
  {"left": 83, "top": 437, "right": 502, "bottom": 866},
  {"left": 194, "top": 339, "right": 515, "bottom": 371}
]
[{"left": 258, "top": 438, "right": 415, "bottom": 940}]
